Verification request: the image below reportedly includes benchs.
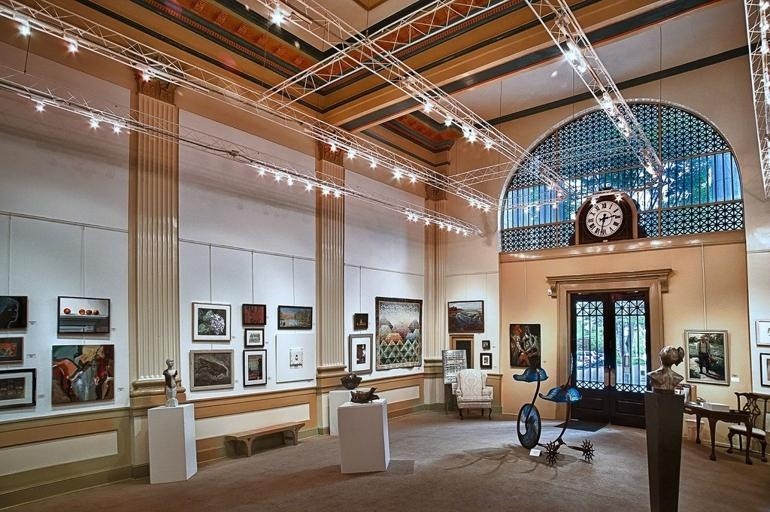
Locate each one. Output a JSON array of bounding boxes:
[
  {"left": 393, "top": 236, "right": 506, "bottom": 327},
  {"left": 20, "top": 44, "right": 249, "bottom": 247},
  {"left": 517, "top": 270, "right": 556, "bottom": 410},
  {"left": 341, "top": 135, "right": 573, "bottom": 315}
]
[{"left": 226, "top": 421, "right": 306, "bottom": 457}]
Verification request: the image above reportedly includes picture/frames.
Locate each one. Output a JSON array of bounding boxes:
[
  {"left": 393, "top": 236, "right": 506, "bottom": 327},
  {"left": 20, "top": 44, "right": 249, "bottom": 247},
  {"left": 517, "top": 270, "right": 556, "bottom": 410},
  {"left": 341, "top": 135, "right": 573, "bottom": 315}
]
[
  {"left": 189, "top": 301, "right": 313, "bottom": 392},
  {"left": 349, "top": 296, "right": 493, "bottom": 374},
  {"left": 683, "top": 319, "right": 770, "bottom": 387},
  {"left": 0, "top": 295, "right": 115, "bottom": 410}
]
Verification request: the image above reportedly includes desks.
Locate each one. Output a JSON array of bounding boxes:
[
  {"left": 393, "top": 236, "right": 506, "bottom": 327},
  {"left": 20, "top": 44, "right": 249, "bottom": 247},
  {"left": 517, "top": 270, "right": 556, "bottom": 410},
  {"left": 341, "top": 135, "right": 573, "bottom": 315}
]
[{"left": 683, "top": 402, "right": 753, "bottom": 463}]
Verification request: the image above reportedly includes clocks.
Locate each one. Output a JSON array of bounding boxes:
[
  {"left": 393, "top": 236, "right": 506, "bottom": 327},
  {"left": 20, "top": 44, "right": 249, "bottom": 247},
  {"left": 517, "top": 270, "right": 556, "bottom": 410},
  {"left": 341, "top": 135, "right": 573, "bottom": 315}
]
[{"left": 575, "top": 190, "right": 638, "bottom": 244}]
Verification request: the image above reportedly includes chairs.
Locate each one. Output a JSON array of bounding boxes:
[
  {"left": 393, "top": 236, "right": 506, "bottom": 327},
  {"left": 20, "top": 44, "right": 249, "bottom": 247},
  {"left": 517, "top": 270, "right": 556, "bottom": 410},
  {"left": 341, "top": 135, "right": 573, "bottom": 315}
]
[
  {"left": 451, "top": 369, "right": 496, "bottom": 420},
  {"left": 726, "top": 391, "right": 770, "bottom": 462}
]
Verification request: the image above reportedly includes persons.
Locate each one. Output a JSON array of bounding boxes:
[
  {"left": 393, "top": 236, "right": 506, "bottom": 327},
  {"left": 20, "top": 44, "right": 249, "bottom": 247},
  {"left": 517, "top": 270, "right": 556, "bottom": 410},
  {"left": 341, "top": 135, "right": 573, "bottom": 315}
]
[
  {"left": 163, "top": 357, "right": 177, "bottom": 389},
  {"left": 512, "top": 325, "right": 524, "bottom": 365},
  {"left": 696, "top": 335, "right": 711, "bottom": 374},
  {"left": 646, "top": 345, "right": 684, "bottom": 392},
  {"left": 523, "top": 325, "right": 541, "bottom": 365}
]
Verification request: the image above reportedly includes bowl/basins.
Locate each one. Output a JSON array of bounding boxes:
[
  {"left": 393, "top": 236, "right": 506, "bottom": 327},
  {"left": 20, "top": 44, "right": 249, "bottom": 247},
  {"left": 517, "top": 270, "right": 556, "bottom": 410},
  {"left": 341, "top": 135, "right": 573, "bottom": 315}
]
[{"left": 340, "top": 374, "right": 362, "bottom": 389}]
